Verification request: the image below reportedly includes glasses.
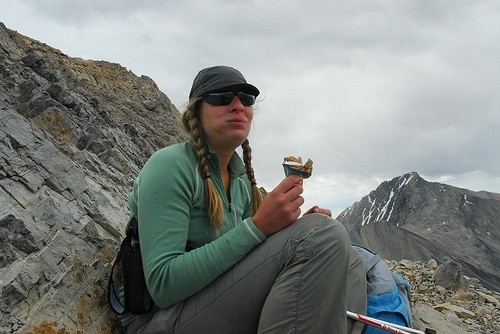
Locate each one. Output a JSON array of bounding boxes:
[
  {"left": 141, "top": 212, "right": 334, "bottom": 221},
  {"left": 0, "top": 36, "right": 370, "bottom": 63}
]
[{"left": 196, "top": 89, "right": 257, "bottom": 106}]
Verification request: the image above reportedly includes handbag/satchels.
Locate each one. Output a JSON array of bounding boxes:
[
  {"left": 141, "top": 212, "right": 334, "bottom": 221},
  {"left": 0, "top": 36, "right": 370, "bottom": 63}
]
[{"left": 107, "top": 217, "right": 195, "bottom": 315}]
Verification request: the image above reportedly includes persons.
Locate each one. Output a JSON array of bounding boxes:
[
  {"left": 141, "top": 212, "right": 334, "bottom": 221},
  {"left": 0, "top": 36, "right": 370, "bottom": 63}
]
[{"left": 113, "top": 65, "right": 367, "bottom": 334}]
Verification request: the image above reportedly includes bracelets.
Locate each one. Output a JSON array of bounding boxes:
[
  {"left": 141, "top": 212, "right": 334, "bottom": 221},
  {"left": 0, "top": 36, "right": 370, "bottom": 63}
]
[{"left": 243, "top": 219, "right": 262, "bottom": 244}]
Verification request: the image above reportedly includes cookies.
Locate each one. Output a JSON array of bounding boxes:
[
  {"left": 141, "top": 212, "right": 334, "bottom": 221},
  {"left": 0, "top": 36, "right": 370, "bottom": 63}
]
[{"left": 283, "top": 155, "right": 302, "bottom": 163}]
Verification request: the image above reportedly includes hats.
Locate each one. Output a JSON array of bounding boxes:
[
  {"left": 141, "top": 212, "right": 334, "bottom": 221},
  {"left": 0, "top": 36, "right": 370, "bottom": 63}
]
[{"left": 189, "top": 66, "right": 260, "bottom": 99}]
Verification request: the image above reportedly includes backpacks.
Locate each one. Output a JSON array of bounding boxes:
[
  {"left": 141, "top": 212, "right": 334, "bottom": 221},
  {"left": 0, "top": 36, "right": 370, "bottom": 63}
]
[{"left": 350, "top": 244, "right": 412, "bottom": 334}]
